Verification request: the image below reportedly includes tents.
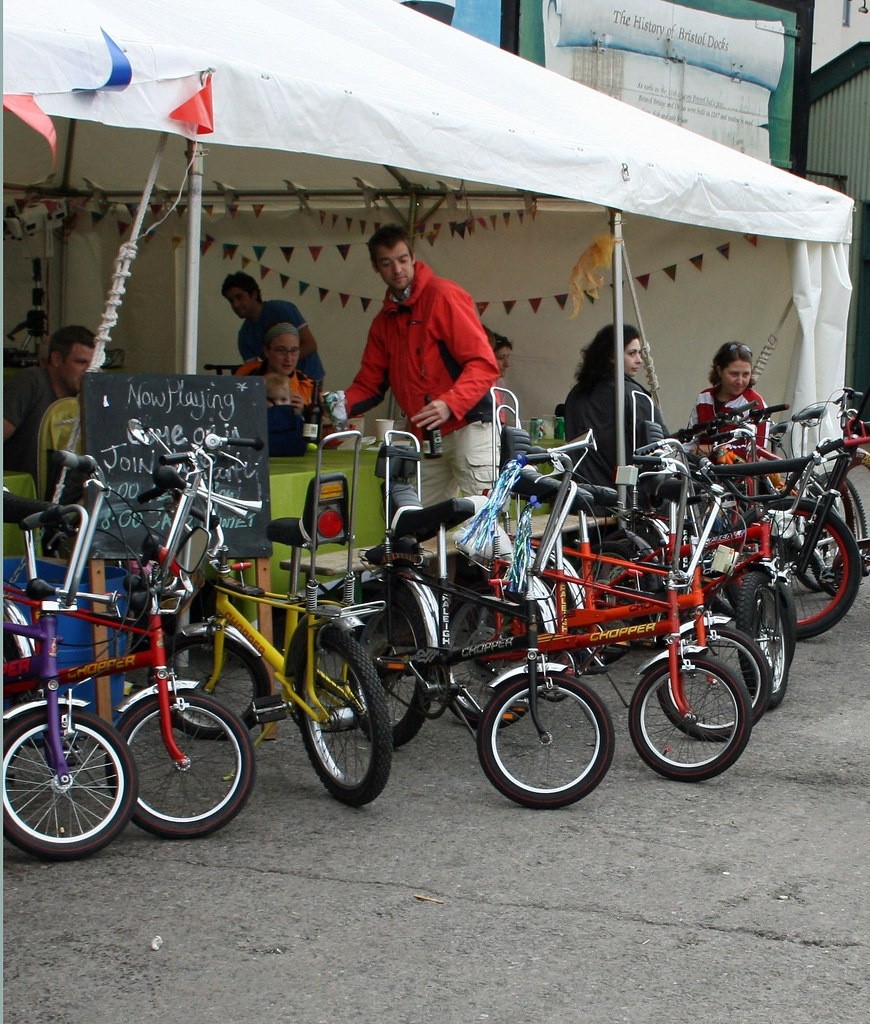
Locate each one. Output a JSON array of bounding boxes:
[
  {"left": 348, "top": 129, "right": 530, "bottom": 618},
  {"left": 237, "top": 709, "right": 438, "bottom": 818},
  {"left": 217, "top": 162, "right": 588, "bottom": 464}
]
[{"left": 3, "top": 0, "right": 850, "bottom": 508}]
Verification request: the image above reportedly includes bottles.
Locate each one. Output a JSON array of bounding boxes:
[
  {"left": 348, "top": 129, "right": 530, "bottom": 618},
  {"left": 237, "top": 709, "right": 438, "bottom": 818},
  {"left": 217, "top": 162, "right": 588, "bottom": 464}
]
[
  {"left": 422, "top": 395, "right": 443, "bottom": 459},
  {"left": 302, "top": 379, "right": 322, "bottom": 442}
]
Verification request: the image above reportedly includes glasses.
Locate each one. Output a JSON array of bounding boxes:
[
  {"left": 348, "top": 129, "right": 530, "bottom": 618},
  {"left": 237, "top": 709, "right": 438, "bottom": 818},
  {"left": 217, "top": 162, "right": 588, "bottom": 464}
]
[
  {"left": 720, "top": 343, "right": 753, "bottom": 358},
  {"left": 269, "top": 345, "right": 301, "bottom": 357}
]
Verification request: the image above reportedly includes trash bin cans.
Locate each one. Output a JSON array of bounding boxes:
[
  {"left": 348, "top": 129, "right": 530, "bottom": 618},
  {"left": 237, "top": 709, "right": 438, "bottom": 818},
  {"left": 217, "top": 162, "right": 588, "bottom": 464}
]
[{"left": 3, "top": 557, "right": 130, "bottom": 741}]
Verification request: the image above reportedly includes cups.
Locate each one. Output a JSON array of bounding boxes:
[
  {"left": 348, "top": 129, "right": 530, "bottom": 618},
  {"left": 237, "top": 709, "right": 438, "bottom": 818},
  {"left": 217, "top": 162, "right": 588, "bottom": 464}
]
[
  {"left": 348, "top": 417, "right": 365, "bottom": 438},
  {"left": 376, "top": 418, "right": 394, "bottom": 442},
  {"left": 541, "top": 415, "right": 556, "bottom": 439},
  {"left": 522, "top": 420, "right": 542, "bottom": 444}
]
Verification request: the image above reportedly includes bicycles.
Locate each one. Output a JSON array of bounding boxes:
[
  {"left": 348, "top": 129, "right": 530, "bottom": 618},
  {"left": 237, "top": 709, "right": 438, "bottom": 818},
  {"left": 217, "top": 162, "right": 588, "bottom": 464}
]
[{"left": 2, "top": 378, "right": 870, "bottom": 863}]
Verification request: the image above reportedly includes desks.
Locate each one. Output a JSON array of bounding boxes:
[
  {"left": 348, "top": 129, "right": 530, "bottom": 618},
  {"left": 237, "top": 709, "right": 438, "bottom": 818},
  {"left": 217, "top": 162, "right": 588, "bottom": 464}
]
[
  {"left": 199, "top": 448, "right": 390, "bottom": 633},
  {"left": 502, "top": 438, "right": 570, "bottom": 519},
  {"left": 4, "top": 471, "right": 43, "bottom": 559}
]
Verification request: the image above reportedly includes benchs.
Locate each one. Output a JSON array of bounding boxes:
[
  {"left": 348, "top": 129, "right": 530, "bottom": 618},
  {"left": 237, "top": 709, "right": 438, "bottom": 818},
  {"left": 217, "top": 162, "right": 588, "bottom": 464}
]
[{"left": 275, "top": 514, "right": 616, "bottom": 604}]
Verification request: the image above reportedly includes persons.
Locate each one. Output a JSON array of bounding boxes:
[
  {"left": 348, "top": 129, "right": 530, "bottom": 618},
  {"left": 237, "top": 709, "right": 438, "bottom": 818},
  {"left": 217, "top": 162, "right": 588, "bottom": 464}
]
[
  {"left": 321, "top": 226, "right": 516, "bottom": 566},
  {"left": 234, "top": 322, "right": 312, "bottom": 446},
  {"left": 3, "top": 326, "right": 97, "bottom": 505},
  {"left": 264, "top": 374, "right": 292, "bottom": 407},
  {"left": 565, "top": 323, "right": 775, "bottom": 651},
  {"left": 221, "top": 271, "right": 326, "bottom": 448}
]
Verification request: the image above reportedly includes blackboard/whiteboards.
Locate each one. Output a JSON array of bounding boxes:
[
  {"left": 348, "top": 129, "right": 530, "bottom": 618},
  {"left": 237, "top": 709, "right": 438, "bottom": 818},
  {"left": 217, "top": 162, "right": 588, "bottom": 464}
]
[{"left": 80, "top": 372, "right": 277, "bottom": 561}]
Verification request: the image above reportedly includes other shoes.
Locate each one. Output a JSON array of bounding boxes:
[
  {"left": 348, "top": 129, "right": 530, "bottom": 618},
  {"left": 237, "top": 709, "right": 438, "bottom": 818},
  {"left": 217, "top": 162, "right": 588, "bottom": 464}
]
[{"left": 392, "top": 632, "right": 417, "bottom": 647}]
